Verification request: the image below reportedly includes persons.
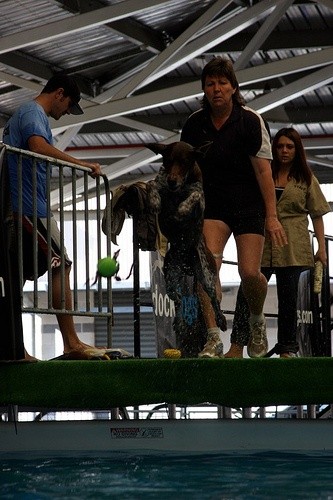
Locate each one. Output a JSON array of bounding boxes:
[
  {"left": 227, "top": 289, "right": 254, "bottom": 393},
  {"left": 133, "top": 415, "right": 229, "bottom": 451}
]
[
  {"left": 180, "top": 57, "right": 288, "bottom": 360},
  {"left": 225, "top": 128, "right": 332, "bottom": 359},
  {"left": 0, "top": 76, "right": 111, "bottom": 360}
]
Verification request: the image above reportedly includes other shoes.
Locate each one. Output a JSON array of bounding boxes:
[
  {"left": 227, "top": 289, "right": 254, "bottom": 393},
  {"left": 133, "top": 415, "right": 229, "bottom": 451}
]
[
  {"left": 198, "top": 333, "right": 224, "bottom": 358},
  {"left": 246, "top": 318, "right": 269, "bottom": 358},
  {"left": 221, "top": 351, "right": 244, "bottom": 358},
  {"left": 280, "top": 352, "right": 298, "bottom": 360}
]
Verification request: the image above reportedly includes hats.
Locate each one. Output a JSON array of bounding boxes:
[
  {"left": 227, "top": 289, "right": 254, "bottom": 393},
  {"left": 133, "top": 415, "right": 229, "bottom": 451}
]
[{"left": 46, "top": 72, "right": 85, "bottom": 115}]
